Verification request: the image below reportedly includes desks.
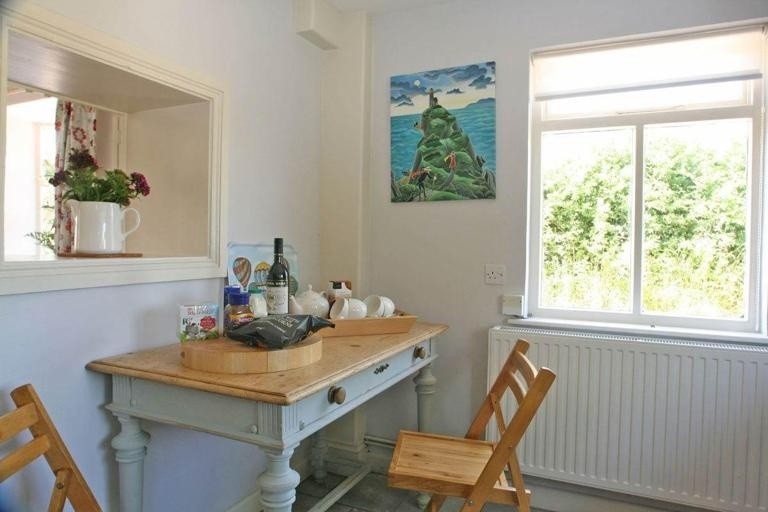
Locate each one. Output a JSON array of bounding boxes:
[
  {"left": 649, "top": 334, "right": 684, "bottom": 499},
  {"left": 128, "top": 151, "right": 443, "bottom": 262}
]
[{"left": 84, "top": 299, "right": 450, "bottom": 512}]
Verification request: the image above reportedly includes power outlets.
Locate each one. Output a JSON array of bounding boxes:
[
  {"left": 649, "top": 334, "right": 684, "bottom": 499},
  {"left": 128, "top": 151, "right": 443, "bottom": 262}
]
[{"left": 485, "top": 263, "right": 507, "bottom": 286}]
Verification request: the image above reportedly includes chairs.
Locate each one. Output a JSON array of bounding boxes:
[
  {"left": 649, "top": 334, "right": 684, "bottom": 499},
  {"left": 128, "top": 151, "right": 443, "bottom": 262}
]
[
  {"left": 0, "top": 383, "right": 100, "bottom": 512},
  {"left": 389, "top": 337, "right": 557, "bottom": 512}
]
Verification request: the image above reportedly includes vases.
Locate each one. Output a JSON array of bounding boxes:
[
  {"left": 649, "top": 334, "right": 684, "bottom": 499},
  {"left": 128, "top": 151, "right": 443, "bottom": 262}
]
[{"left": 67, "top": 199, "right": 140, "bottom": 256}]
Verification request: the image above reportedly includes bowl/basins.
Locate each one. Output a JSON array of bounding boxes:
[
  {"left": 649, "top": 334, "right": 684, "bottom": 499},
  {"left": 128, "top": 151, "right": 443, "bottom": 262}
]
[{"left": 328, "top": 296, "right": 395, "bottom": 321}]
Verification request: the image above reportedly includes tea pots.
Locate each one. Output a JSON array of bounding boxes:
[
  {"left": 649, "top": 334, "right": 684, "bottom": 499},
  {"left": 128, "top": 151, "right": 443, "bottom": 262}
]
[{"left": 288, "top": 284, "right": 329, "bottom": 319}]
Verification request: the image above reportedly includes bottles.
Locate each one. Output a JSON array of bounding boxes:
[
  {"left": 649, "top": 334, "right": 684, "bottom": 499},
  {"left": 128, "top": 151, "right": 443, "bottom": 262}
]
[
  {"left": 223, "top": 285, "right": 240, "bottom": 309},
  {"left": 225, "top": 293, "right": 253, "bottom": 335},
  {"left": 248, "top": 288, "right": 267, "bottom": 322},
  {"left": 264, "top": 237, "right": 289, "bottom": 315}
]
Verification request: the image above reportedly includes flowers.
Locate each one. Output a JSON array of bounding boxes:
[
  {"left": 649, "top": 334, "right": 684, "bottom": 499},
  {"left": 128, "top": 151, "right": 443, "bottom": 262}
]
[{"left": 22, "top": 146, "right": 151, "bottom": 250}]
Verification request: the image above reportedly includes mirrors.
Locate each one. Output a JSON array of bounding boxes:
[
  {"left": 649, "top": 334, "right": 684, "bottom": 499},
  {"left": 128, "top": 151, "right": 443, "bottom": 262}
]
[{"left": 0, "top": 1, "right": 230, "bottom": 296}]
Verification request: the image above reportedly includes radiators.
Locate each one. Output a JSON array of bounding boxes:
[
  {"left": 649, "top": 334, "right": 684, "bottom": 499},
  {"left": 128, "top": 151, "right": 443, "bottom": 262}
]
[{"left": 485, "top": 327, "right": 768, "bottom": 512}]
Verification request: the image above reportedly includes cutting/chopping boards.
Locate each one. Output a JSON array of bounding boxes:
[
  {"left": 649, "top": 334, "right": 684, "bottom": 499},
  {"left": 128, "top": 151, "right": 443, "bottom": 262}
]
[{"left": 179, "top": 336, "right": 323, "bottom": 374}]
